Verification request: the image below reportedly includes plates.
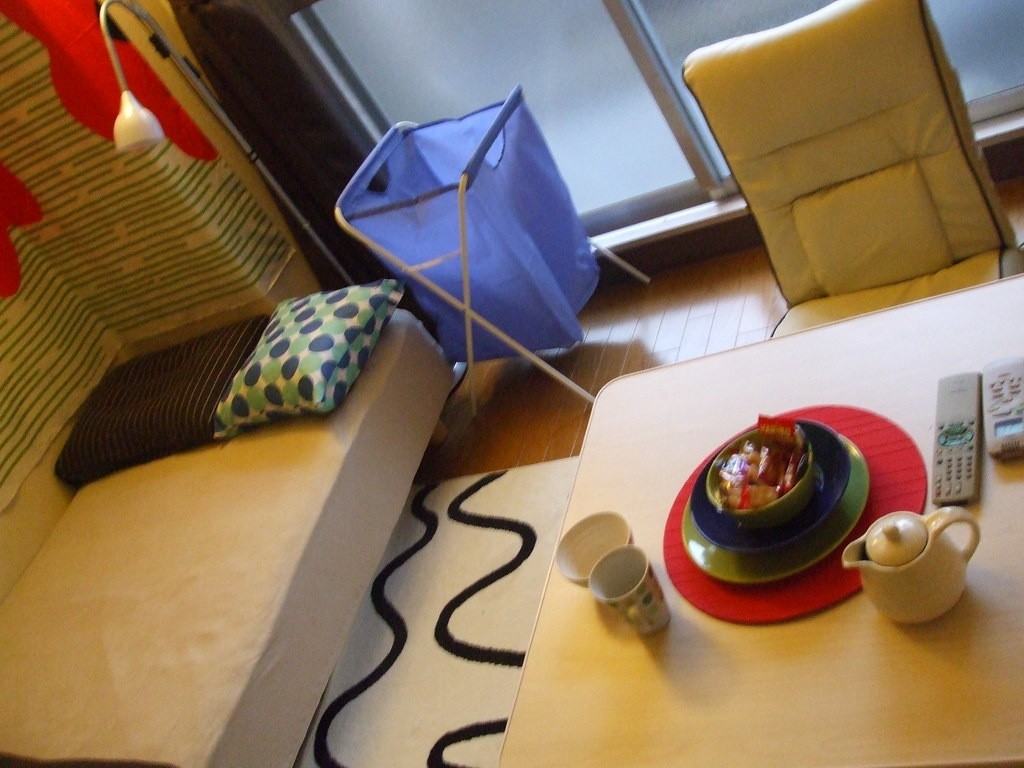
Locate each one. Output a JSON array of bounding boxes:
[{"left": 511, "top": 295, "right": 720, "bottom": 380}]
[{"left": 682, "top": 420, "right": 869, "bottom": 584}]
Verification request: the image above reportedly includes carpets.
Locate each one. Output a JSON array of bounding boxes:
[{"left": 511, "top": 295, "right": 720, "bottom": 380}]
[{"left": 294, "top": 455, "right": 577, "bottom": 768}]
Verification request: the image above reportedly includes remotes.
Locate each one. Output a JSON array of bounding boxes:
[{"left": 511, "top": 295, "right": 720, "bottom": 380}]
[
  {"left": 983, "top": 357, "right": 1024, "bottom": 461},
  {"left": 931, "top": 371, "right": 983, "bottom": 507}
]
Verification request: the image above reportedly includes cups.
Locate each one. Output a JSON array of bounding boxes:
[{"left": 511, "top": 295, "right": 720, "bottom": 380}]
[{"left": 555, "top": 511, "right": 672, "bottom": 637}]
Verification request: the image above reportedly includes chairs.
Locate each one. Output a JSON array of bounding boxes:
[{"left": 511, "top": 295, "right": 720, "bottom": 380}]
[{"left": 682, "top": 0, "right": 1024, "bottom": 338}]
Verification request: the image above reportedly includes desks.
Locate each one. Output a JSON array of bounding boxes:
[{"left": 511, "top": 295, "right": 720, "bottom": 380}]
[{"left": 497, "top": 274, "right": 1024, "bottom": 768}]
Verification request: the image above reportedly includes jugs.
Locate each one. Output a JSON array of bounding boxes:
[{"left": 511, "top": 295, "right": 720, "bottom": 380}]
[{"left": 841, "top": 505, "right": 981, "bottom": 625}]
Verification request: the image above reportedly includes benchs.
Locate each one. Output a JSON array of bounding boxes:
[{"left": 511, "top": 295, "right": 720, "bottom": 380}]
[{"left": 0, "top": 311, "right": 455, "bottom": 768}]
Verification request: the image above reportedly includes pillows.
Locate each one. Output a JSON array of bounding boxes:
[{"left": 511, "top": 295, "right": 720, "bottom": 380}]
[
  {"left": 54, "top": 313, "right": 271, "bottom": 490},
  {"left": 214, "top": 276, "right": 405, "bottom": 439}
]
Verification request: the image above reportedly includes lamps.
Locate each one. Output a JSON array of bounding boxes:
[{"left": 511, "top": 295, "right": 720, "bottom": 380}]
[{"left": 99, "top": 0, "right": 356, "bottom": 286}]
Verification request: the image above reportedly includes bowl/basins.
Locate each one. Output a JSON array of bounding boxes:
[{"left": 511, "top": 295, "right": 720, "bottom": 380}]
[{"left": 707, "top": 426, "right": 815, "bottom": 528}]
